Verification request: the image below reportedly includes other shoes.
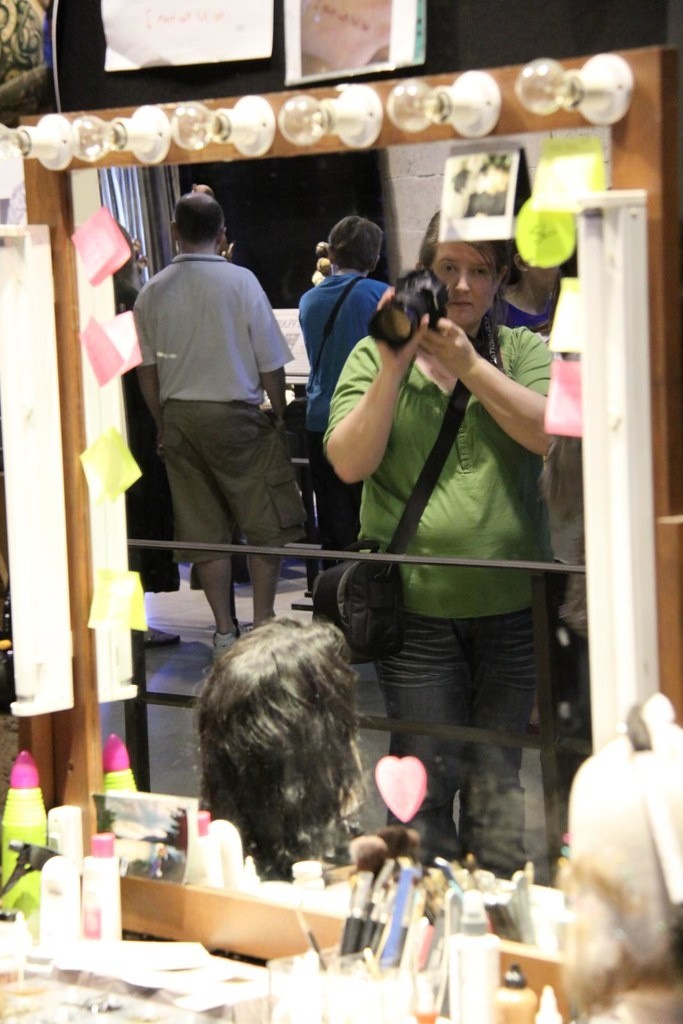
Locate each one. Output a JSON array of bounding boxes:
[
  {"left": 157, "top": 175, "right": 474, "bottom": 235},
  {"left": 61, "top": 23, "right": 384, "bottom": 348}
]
[{"left": 214, "top": 622, "right": 254, "bottom": 663}]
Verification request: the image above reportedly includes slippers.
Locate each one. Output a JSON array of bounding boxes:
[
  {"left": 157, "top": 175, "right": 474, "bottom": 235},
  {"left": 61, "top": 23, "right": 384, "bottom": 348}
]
[{"left": 143, "top": 626, "right": 180, "bottom": 648}]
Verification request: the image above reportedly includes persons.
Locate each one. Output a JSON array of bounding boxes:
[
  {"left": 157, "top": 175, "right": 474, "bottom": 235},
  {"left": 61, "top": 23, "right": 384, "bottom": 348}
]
[
  {"left": 324, "top": 212, "right": 564, "bottom": 881},
  {"left": 490, "top": 237, "right": 563, "bottom": 340},
  {"left": 109, "top": 221, "right": 179, "bottom": 647},
  {"left": 132, "top": 191, "right": 293, "bottom": 666},
  {"left": 196, "top": 616, "right": 366, "bottom": 882},
  {"left": 299, "top": 218, "right": 404, "bottom": 571}
]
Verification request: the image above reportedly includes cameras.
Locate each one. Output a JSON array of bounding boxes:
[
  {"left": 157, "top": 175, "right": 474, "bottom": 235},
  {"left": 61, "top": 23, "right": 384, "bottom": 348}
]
[{"left": 368, "top": 267, "right": 449, "bottom": 348}]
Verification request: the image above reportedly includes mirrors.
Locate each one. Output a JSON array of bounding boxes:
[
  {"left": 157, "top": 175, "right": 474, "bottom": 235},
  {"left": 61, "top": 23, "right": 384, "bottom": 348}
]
[{"left": 16, "top": 46, "right": 683, "bottom": 1024}]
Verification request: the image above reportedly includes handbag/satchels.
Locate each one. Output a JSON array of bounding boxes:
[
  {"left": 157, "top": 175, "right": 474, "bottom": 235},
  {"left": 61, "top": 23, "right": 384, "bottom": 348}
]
[
  {"left": 283, "top": 397, "right": 305, "bottom": 432},
  {"left": 313, "top": 555, "right": 403, "bottom": 663}
]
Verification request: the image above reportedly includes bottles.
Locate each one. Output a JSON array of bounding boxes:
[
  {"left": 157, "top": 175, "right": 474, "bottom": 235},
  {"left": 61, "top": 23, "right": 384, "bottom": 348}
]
[
  {"left": 3, "top": 752, "right": 47, "bottom": 945},
  {"left": 200, "top": 810, "right": 262, "bottom": 896},
  {"left": 293, "top": 861, "right": 325, "bottom": 908},
  {"left": 85, "top": 832, "right": 123, "bottom": 938},
  {"left": 102, "top": 733, "right": 137, "bottom": 793},
  {"left": 45, "top": 805, "right": 84, "bottom": 882},
  {"left": 449, "top": 890, "right": 499, "bottom": 1024},
  {"left": 43, "top": 859, "right": 82, "bottom": 953}
]
